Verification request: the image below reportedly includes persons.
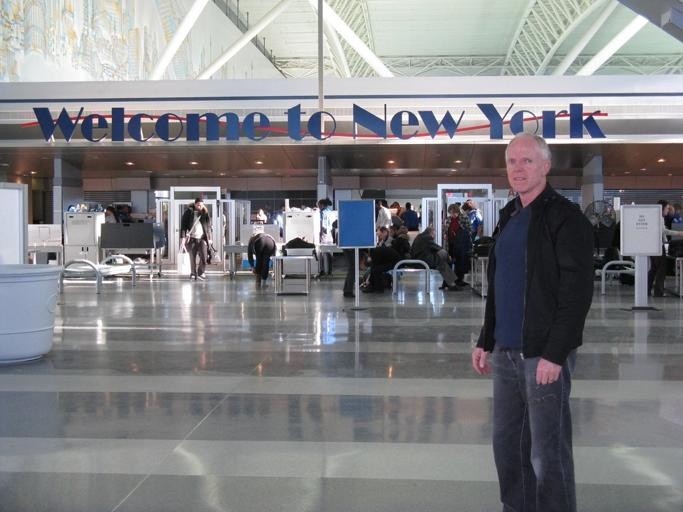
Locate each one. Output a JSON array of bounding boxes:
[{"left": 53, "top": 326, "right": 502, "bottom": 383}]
[
  {"left": 179, "top": 196, "right": 210, "bottom": 281},
  {"left": 247, "top": 232, "right": 277, "bottom": 287},
  {"left": 472, "top": 133, "right": 595, "bottom": 512},
  {"left": 586, "top": 199, "right": 683, "bottom": 298},
  {"left": 317, "top": 195, "right": 482, "bottom": 299},
  {"left": 104, "top": 204, "right": 135, "bottom": 223}
]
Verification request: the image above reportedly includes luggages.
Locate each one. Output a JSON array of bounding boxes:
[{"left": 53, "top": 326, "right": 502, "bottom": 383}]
[{"left": 472, "top": 236, "right": 492, "bottom": 257}]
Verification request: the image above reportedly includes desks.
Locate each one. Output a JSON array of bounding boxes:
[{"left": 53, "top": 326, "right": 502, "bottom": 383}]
[
  {"left": 273, "top": 255, "right": 313, "bottom": 296},
  {"left": 284, "top": 248, "right": 315, "bottom": 275},
  {"left": 469, "top": 255, "right": 490, "bottom": 299}
]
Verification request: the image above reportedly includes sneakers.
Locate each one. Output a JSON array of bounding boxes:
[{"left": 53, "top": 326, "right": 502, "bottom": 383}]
[
  {"left": 189, "top": 272, "right": 209, "bottom": 281},
  {"left": 439, "top": 280, "right": 466, "bottom": 292}
]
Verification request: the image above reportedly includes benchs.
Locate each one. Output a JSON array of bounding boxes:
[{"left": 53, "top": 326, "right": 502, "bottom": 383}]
[
  {"left": 60, "top": 253, "right": 136, "bottom": 294},
  {"left": 595, "top": 249, "right": 636, "bottom": 294},
  {"left": 388, "top": 259, "right": 431, "bottom": 295}
]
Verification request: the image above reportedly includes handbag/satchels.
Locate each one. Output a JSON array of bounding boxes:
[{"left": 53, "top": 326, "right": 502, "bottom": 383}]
[
  {"left": 176, "top": 243, "right": 191, "bottom": 276},
  {"left": 283, "top": 237, "right": 316, "bottom": 249}
]
